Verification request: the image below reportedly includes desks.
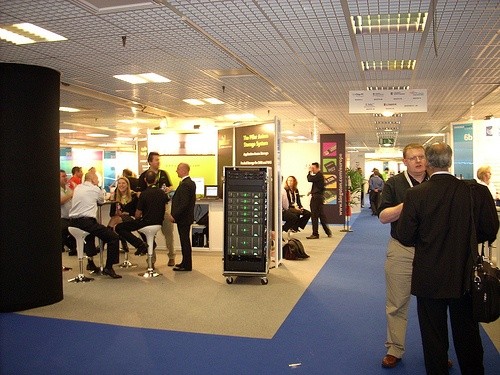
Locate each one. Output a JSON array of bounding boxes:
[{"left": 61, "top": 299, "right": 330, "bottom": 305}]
[{"left": 89, "top": 200, "right": 119, "bottom": 276}]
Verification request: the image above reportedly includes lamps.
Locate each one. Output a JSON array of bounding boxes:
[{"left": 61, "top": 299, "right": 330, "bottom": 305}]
[
  {"left": 184, "top": 122, "right": 201, "bottom": 130},
  {"left": 154, "top": 120, "right": 165, "bottom": 130}
]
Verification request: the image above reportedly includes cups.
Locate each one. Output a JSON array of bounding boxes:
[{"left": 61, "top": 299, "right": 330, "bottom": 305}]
[{"left": 104, "top": 193, "right": 111, "bottom": 202}]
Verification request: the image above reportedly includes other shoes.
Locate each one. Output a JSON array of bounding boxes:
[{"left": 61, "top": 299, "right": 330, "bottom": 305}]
[
  {"left": 95, "top": 247, "right": 104, "bottom": 252},
  {"left": 167, "top": 259, "right": 175, "bottom": 266},
  {"left": 135, "top": 243, "right": 148, "bottom": 255},
  {"left": 102, "top": 265, "right": 122, "bottom": 278},
  {"left": 448, "top": 360, "right": 452, "bottom": 368},
  {"left": 306, "top": 234, "right": 318, "bottom": 239},
  {"left": 382, "top": 354, "right": 402, "bottom": 368},
  {"left": 174, "top": 267, "right": 192, "bottom": 271},
  {"left": 328, "top": 231, "right": 332, "bottom": 238},
  {"left": 86, "top": 264, "right": 99, "bottom": 272},
  {"left": 176, "top": 263, "right": 184, "bottom": 267}
]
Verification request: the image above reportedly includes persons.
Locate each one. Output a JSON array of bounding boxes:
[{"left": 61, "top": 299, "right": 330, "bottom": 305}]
[
  {"left": 377, "top": 142, "right": 454, "bottom": 368},
  {"left": 67, "top": 172, "right": 122, "bottom": 279},
  {"left": 477, "top": 166, "right": 495, "bottom": 248},
  {"left": 68, "top": 167, "right": 99, "bottom": 189},
  {"left": 306, "top": 162, "right": 331, "bottom": 239},
  {"left": 397, "top": 141, "right": 500, "bottom": 375},
  {"left": 284, "top": 176, "right": 311, "bottom": 233},
  {"left": 139, "top": 152, "right": 174, "bottom": 270},
  {"left": 367, "top": 168, "right": 388, "bottom": 216},
  {"left": 282, "top": 176, "right": 299, "bottom": 232},
  {"left": 357, "top": 168, "right": 364, "bottom": 208},
  {"left": 59, "top": 170, "right": 73, "bottom": 271},
  {"left": 96, "top": 176, "right": 139, "bottom": 252},
  {"left": 114, "top": 169, "right": 139, "bottom": 191},
  {"left": 171, "top": 162, "right": 196, "bottom": 271},
  {"left": 114, "top": 170, "right": 170, "bottom": 256}
]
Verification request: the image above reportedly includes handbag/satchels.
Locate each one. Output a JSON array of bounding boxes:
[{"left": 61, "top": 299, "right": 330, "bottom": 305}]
[
  {"left": 471, "top": 256, "right": 500, "bottom": 323},
  {"left": 283, "top": 244, "right": 297, "bottom": 260},
  {"left": 288, "top": 239, "right": 309, "bottom": 258}
]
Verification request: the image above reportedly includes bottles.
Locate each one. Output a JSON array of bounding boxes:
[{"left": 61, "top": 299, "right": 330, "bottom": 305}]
[{"left": 162, "top": 184, "right": 166, "bottom": 189}]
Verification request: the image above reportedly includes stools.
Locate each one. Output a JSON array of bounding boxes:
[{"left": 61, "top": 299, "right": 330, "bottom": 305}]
[
  {"left": 68, "top": 226, "right": 95, "bottom": 284},
  {"left": 137, "top": 225, "right": 163, "bottom": 278}
]
